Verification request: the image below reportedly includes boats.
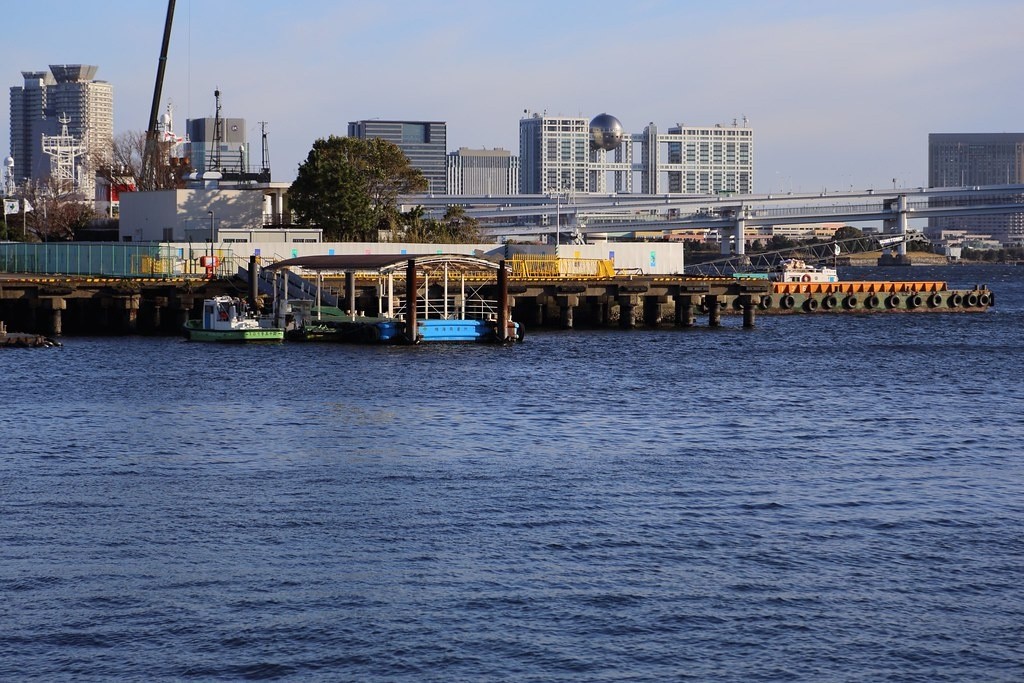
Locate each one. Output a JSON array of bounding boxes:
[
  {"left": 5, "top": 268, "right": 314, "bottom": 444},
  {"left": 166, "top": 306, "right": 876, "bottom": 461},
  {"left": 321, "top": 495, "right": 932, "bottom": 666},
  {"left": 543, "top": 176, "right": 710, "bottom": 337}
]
[{"left": 183, "top": 295, "right": 285, "bottom": 344}]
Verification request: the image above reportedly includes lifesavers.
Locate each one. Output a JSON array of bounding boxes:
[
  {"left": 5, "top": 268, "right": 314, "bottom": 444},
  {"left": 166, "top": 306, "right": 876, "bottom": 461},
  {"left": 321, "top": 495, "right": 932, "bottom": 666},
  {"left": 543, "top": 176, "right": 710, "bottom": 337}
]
[
  {"left": 761, "top": 295, "right": 773, "bottom": 309},
  {"left": 802, "top": 274, "right": 811, "bottom": 283},
  {"left": 783, "top": 295, "right": 795, "bottom": 308},
  {"left": 846, "top": 296, "right": 858, "bottom": 308},
  {"left": 808, "top": 298, "right": 818, "bottom": 311},
  {"left": 967, "top": 293, "right": 978, "bottom": 307},
  {"left": 931, "top": 294, "right": 943, "bottom": 307},
  {"left": 911, "top": 295, "right": 923, "bottom": 307},
  {"left": 826, "top": 295, "right": 838, "bottom": 308},
  {"left": 738, "top": 298, "right": 745, "bottom": 309},
  {"left": 979, "top": 294, "right": 990, "bottom": 306},
  {"left": 868, "top": 296, "right": 880, "bottom": 308},
  {"left": 951, "top": 294, "right": 964, "bottom": 307},
  {"left": 517, "top": 322, "right": 525, "bottom": 340},
  {"left": 889, "top": 295, "right": 901, "bottom": 307},
  {"left": 720, "top": 301, "right": 728, "bottom": 310}
]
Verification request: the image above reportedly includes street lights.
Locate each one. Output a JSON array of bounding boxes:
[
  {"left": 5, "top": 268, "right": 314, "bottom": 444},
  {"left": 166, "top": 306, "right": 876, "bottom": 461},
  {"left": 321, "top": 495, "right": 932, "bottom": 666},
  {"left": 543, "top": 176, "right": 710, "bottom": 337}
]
[{"left": 208, "top": 210, "right": 214, "bottom": 277}]
